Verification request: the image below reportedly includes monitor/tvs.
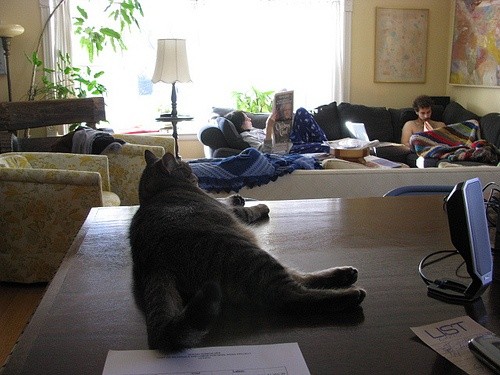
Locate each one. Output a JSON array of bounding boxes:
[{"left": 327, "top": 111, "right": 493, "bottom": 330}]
[{"left": 427, "top": 176, "right": 494, "bottom": 302}]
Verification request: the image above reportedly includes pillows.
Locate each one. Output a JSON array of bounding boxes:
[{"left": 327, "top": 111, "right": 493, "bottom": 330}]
[{"left": 208, "top": 96, "right": 500, "bottom": 150}]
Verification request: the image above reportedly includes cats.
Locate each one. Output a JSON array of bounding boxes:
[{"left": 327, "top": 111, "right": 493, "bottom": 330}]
[{"left": 125, "top": 148, "right": 367, "bottom": 357}]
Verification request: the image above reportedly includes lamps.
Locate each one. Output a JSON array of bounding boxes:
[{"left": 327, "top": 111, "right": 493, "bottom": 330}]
[
  {"left": 151, "top": 39, "right": 192, "bottom": 160},
  {"left": 0, "top": 24, "right": 24, "bottom": 136}
]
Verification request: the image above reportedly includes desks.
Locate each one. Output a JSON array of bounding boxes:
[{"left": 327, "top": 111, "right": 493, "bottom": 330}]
[{"left": 0, "top": 197, "right": 500, "bottom": 375}]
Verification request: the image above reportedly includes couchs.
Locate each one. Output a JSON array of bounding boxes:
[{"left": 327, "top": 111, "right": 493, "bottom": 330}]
[
  {"left": 195, "top": 94, "right": 500, "bottom": 159},
  {"left": 74, "top": 125, "right": 175, "bottom": 206},
  {"left": 0, "top": 153, "right": 121, "bottom": 284}
]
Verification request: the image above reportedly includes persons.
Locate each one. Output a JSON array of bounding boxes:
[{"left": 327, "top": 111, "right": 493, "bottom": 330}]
[
  {"left": 401, "top": 95, "right": 445, "bottom": 146},
  {"left": 226, "top": 108, "right": 330, "bottom": 155}
]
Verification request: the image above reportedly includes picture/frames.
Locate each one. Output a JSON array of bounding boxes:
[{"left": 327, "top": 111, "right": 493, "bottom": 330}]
[
  {"left": 447, "top": 0, "right": 500, "bottom": 88},
  {"left": 373, "top": 6, "right": 430, "bottom": 84}
]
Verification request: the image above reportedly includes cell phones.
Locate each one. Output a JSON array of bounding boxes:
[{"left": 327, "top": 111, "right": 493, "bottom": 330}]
[{"left": 467, "top": 334, "right": 500, "bottom": 375}]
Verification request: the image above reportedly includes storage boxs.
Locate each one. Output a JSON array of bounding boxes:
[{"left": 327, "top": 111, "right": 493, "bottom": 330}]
[{"left": 330, "top": 148, "right": 369, "bottom": 159}]
